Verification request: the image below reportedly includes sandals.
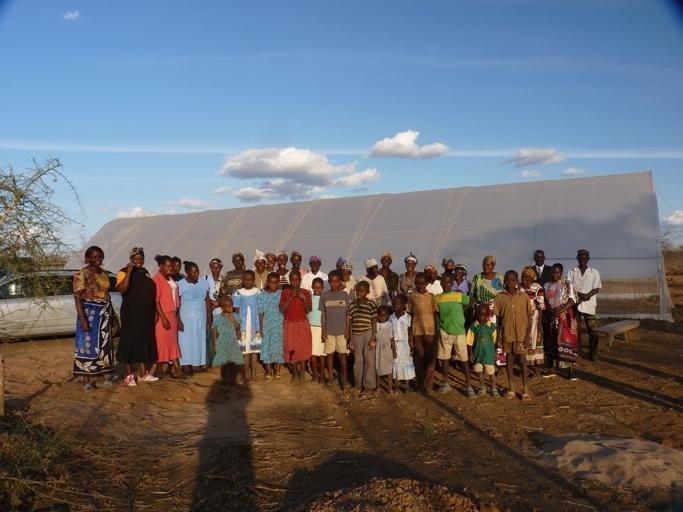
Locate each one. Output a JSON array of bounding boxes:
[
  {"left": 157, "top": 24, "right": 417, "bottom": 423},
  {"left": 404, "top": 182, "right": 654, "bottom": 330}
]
[
  {"left": 263, "top": 375, "right": 272, "bottom": 385},
  {"left": 273, "top": 375, "right": 281, "bottom": 383}
]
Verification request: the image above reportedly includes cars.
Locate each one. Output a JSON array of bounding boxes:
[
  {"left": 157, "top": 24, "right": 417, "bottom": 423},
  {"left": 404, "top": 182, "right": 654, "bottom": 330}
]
[{"left": 0, "top": 269, "right": 124, "bottom": 340}]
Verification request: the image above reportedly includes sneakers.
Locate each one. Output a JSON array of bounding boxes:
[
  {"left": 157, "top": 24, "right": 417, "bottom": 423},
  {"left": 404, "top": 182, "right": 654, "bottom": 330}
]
[
  {"left": 124, "top": 374, "right": 136, "bottom": 386},
  {"left": 542, "top": 371, "right": 556, "bottom": 378},
  {"left": 491, "top": 388, "right": 499, "bottom": 397},
  {"left": 466, "top": 388, "right": 476, "bottom": 398},
  {"left": 569, "top": 374, "right": 577, "bottom": 381},
  {"left": 477, "top": 387, "right": 486, "bottom": 395},
  {"left": 137, "top": 373, "right": 158, "bottom": 381},
  {"left": 438, "top": 385, "right": 450, "bottom": 393}
]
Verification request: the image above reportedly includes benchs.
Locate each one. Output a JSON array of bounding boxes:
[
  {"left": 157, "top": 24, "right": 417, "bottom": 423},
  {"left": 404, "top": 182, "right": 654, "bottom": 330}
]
[{"left": 592, "top": 319, "right": 641, "bottom": 350}]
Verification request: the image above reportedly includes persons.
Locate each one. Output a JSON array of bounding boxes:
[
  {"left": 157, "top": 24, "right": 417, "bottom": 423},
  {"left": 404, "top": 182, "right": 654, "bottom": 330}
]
[
  {"left": 116, "top": 246, "right": 600, "bottom": 402},
  {"left": 73, "top": 246, "right": 117, "bottom": 392}
]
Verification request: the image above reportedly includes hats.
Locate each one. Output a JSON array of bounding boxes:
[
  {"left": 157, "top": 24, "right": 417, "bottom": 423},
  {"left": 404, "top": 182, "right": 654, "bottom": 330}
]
[
  {"left": 276, "top": 250, "right": 288, "bottom": 260},
  {"left": 381, "top": 251, "right": 391, "bottom": 259},
  {"left": 366, "top": 258, "right": 377, "bottom": 268},
  {"left": 291, "top": 250, "right": 300, "bottom": 259},
  {"left": 338, "top": 260, "right": 353, "bottom": 273},
  {"left": 254, "top": 249, "right": 268, "bottom": 264},
  {"left": 425, "top": 264, "right": 437, "bottom": 274},
  {"left": 309, "top": 255, "right": 321, "bottom": 262}
]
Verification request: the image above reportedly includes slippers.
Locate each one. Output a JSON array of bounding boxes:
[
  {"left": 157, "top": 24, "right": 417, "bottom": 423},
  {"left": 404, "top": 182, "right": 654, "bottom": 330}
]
[
  {"left": 96, "top": 380, "right": 114, "bottom": 386},
  {"left": 521, "top": 394, "right": 531, "bottom": 400},
  {"left": 504, "top": 391, "right": 515, "bottom": 400},
  {"left": 84, "top": 383, "right": 94, "bottom": 392}
]
